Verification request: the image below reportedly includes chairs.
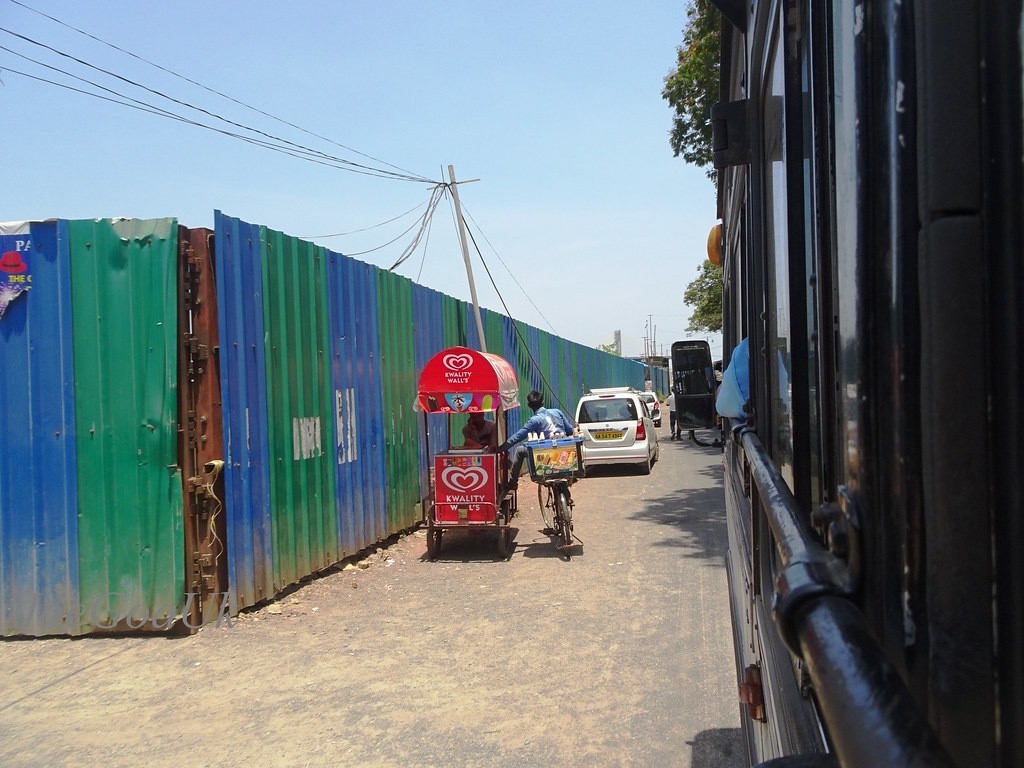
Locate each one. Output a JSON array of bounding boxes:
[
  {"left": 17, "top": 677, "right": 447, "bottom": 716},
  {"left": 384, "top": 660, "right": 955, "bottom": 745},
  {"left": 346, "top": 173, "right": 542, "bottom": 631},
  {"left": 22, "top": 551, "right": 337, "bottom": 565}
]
[
  {"left": 595, "top": 407, "right": 608, "bottom": 420},
  {"left": 619, "top": 406, "right": 630, "bottom": 419}
]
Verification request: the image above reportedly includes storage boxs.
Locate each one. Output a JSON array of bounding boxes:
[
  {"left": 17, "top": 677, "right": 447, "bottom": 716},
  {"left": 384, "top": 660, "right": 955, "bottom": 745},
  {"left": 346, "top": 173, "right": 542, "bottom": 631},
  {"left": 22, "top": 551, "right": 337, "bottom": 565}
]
[{"left": 523, "top": 436, "right": 586, "bottom": 482}]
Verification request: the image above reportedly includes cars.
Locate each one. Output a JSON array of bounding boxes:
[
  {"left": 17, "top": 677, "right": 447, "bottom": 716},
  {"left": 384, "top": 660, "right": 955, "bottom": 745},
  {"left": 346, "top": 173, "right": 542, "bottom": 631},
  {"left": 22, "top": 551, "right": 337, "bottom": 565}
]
[
  {"left": 574, "top": 387, "right": 659, "bottom": 476},
  {"left": 637, "top": 393, "right": 662, "bottom": 426}
]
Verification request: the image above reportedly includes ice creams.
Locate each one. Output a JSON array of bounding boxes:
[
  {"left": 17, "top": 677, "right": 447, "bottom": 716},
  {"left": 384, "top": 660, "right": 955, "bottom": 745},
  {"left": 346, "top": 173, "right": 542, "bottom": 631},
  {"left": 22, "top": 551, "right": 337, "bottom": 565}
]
[{"left": 452, "top": 395, "right": 465, "bottom": 412}]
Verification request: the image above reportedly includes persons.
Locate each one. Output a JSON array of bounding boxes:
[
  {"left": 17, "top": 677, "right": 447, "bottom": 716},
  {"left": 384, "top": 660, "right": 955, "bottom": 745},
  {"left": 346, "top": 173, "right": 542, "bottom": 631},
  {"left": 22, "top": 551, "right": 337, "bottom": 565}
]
[
  {"left": 666, "top": 386, "right": 683, "bottom": 440},
  {"left": 715, "top": 338, "right": 749, "bottom": 419},
  {"left": 488, "top": 391, "right": 576, "bottom": 505},
  {"left": 461, "top": 412, "right": 496, "bottom": 446}
]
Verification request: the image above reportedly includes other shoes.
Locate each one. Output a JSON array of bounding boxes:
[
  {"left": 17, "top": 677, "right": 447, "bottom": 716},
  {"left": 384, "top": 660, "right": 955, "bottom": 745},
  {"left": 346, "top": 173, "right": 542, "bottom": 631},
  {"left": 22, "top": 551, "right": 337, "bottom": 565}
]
[
  {"left": 508, "top": 482, "right": 518, "bottom": 489},
  {"left": 677, "top": 437, "right": 682, "bottom": 440},
  {"left": 671, "top": 433, "right": 675, "bottom": 440},
  {"left": 567, "top": 499, "right": 574, "bottom": 506}
]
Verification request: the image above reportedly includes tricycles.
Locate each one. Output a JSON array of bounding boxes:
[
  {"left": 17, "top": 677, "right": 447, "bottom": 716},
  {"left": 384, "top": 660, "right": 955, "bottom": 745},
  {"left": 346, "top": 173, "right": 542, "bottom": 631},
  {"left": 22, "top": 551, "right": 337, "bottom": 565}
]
[{"left": 413, "top": 345, "right": 520, "bottom": 559}]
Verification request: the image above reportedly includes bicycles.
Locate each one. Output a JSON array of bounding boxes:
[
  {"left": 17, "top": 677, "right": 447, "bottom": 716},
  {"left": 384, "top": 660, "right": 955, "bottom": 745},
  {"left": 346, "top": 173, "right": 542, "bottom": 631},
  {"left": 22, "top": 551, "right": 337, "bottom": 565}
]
[{"left": 523, "top": 430, "right": 585, "bottom": 559}]
